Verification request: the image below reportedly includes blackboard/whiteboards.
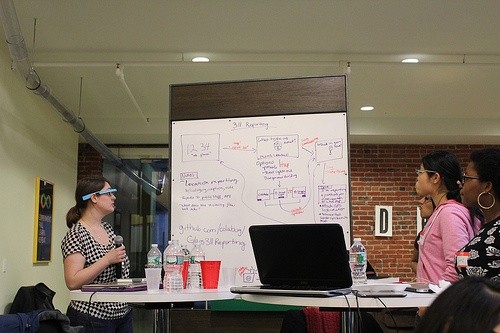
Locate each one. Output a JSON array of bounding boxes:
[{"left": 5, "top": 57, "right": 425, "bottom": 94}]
[{"left": 171, "top": 112, "right": 352, "bottom": 287}]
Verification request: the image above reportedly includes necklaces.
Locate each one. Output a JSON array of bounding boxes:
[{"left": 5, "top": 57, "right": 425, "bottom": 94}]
[
  {"left": 437, "top": 193, "right": 446, "bottom": 207},
  {"left": 82, "top": 219, "right": 91, "bottom": 228}
]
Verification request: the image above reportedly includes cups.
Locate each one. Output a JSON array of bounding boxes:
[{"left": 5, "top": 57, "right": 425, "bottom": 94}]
[
  {"left": 181, "top": 259, "right": 190, "bottom": 289},
  {"left": 199, "top": 260, "right": 221, "bottom": 292},
  {"left": 144, "top": 268, "right": 162, "bottom": 295}
]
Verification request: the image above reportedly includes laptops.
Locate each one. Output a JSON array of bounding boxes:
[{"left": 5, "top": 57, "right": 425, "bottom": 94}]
[{"left": 230, "top": 224, "right": 353, "bottom": 298}]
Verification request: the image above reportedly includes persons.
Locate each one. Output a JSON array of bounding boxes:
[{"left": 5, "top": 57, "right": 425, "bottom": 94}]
[
  {"left": 412, "top": 148, "right": 500, "bottom": 333},
  {"left": 60, "top": 176, "right": 133, "bottom": 333}
]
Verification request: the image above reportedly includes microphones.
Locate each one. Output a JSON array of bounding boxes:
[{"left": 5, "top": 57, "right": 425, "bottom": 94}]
[{"left": 114, "top": 236, "right": 124, "bottom": 278}]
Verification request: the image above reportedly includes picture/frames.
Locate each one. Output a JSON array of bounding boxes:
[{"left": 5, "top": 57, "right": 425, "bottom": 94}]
[{"left": 33, "top": 176, "right": 56, "bottom": 265}]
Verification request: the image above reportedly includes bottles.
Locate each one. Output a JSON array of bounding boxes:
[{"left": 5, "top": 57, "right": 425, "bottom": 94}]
[
  {"left": 180, "top": 243, "right": 190, "bottom": 260},
  {"left": 146, "top": 244, "right": 162, "bottom": 268},
  {"left": 349, "top": 237, "right": 368, "bottom": 286},
  {"left": 163, "top": 241, "right": 184, "bottom": 295},
  {"left": 188, "top": 243, "right": 206, "bottom": 289}
]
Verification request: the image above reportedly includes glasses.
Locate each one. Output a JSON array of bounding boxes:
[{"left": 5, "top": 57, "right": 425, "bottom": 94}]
[
  {"left": 415, "top": 167, "right": 437, "bottom": 174},
  {"left": 461, "top": 173, "right": 478, "bottom": 185}
]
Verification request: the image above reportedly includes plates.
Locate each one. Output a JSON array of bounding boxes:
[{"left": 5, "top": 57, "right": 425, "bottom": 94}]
[{"left": 352, "top": 284, "right": 409, "bottom": 292}]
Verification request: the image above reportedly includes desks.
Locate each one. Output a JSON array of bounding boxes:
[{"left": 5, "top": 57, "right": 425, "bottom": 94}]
[{"left": 67, "top": 282, "right": 439, "bottom": 333}]
